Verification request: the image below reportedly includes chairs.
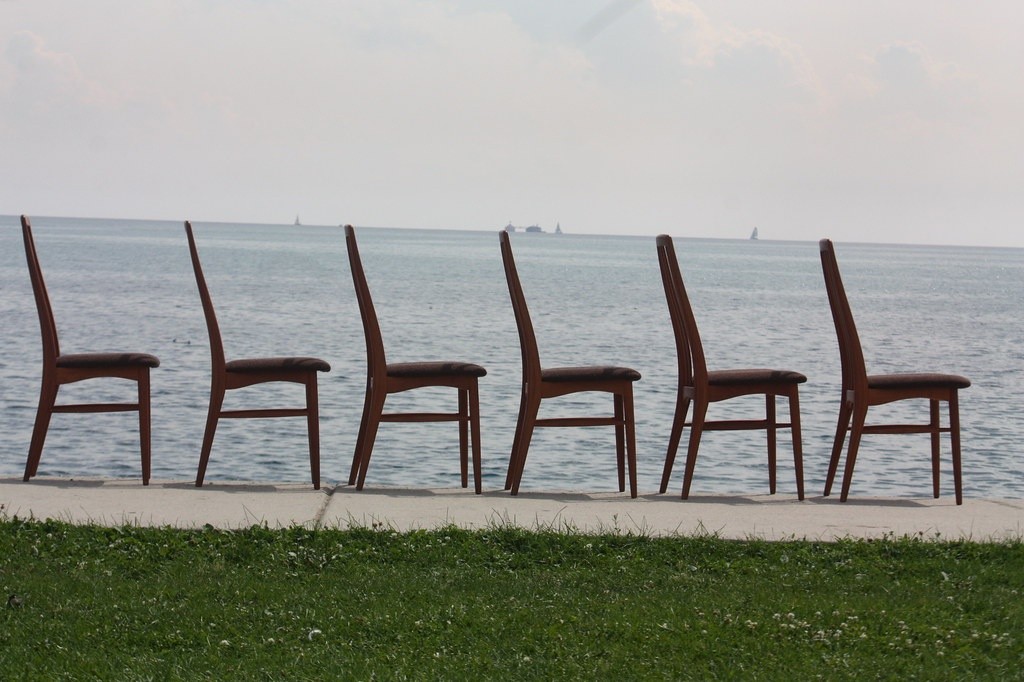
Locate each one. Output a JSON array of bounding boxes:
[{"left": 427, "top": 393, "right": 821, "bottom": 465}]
[
  {"left": 656, "top": 234, "right": 809, "bottom": 503},
  {"left": 344, "top": 225, "right": 487, "bottom": 496},
  {"left": 498, "top": 231, "right": 642, "bottom": 499},
  {"left": 184, "top": 219, "right": 331, "bottom": 490},
  {"left": 21, "top": 215, "right": 160, "bottom": 487},
  {"left": 819, "top": 238, "right": 972, "bottom": 505}
]
